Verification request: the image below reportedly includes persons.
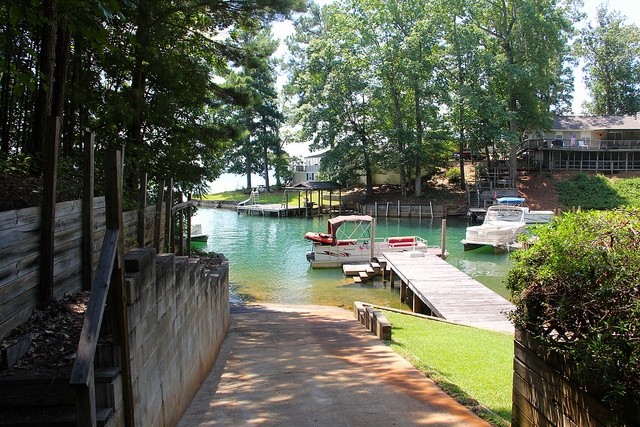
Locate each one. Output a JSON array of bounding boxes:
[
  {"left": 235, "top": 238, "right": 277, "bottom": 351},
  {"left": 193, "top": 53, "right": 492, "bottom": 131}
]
[
  {"left": 473, "top": 212, "right": 476, "bottom": 222},
  {"left": 431, "top": 182, "right": 434, "bottom": 187},
  {"left": 428, "top": 182, "right": 431, "bottom": 186}
]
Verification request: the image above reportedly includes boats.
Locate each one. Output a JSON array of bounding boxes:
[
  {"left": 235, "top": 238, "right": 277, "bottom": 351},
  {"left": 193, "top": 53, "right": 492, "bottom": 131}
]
[
  {"left": 493, "top": 192, "right": 555, "bottom": 223},
  {"left": 460, "top": 204, "right": 526, "bottom": 254},
  {"left": 304, "top": 214, "right": 450, "bottom": 269},
  {"left": 187, "top": 222, "right": 210, "bottom": 251}
]
